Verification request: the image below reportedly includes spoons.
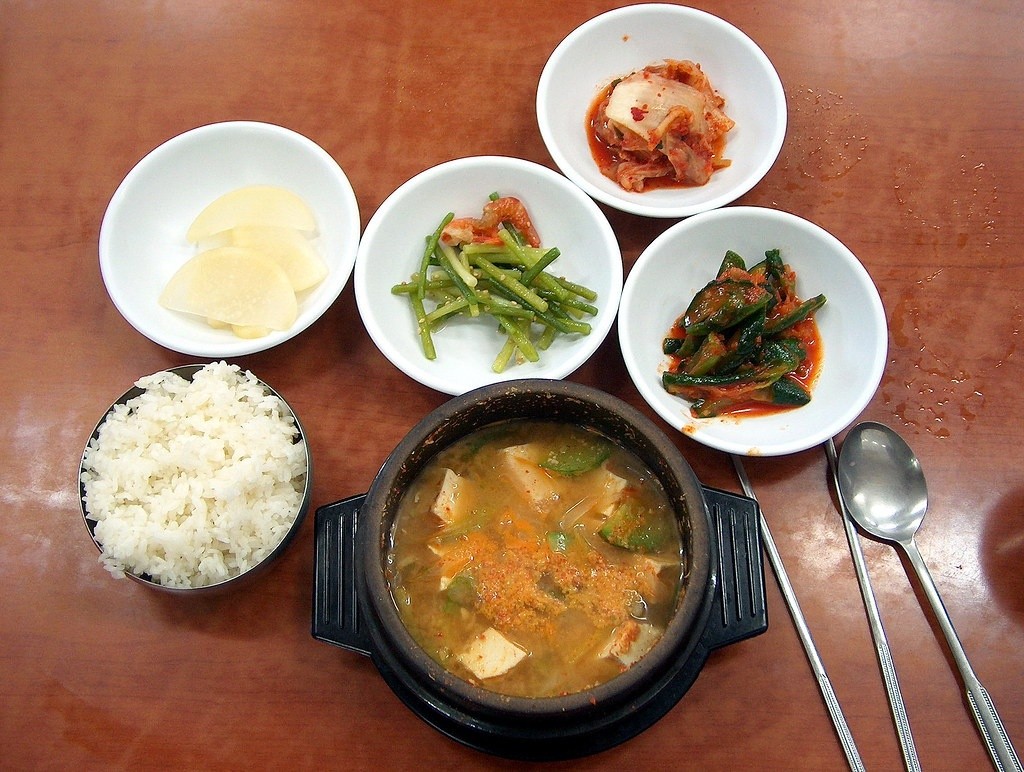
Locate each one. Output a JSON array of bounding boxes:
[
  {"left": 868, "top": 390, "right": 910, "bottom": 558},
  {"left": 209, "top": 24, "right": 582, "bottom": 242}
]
[{"left": 836, "top": 418, "right": 1024, "bottom": 772}]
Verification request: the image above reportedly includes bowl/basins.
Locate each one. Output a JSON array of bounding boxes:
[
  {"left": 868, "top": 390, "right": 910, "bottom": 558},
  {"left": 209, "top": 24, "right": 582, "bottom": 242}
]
[
  {"left": 306, "top": 380, "right": 766, "bottom": 765},
  {"left": 98, "top": 121, "right": 361, "bottom": 359},
  {"left": 535, "top": 4, "right": 786, "bottom": 221},
  {"left": 617, "top": 203, "right": 889, "bottom": 456},
  {"left": 75, "top": 363, "right": 312, "bottom": 601},
  {"left": 353, "top": 152, "right": 623, "bottom": 398}
]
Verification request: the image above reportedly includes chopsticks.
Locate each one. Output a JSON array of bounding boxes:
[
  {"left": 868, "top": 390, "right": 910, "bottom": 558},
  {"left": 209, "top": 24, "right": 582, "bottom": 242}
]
[{"left": 727, "top": 430, "right": 924, "bottom": 772}]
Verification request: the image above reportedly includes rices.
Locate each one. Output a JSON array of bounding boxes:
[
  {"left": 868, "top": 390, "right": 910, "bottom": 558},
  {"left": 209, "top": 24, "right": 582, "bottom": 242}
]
[{"left": 79, "top": 360, "right": 307, "bottom": 590}]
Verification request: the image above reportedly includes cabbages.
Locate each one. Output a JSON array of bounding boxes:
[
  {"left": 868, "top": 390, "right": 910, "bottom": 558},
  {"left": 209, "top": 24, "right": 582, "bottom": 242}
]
[{"left": 594, "top": 59, "right": 735, "bottom": 193}]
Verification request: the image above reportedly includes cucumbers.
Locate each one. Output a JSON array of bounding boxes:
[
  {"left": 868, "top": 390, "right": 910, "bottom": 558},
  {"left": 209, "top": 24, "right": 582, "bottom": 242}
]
[{"left": 662, "top": 249, "right": 828, "bottom": 418}]
